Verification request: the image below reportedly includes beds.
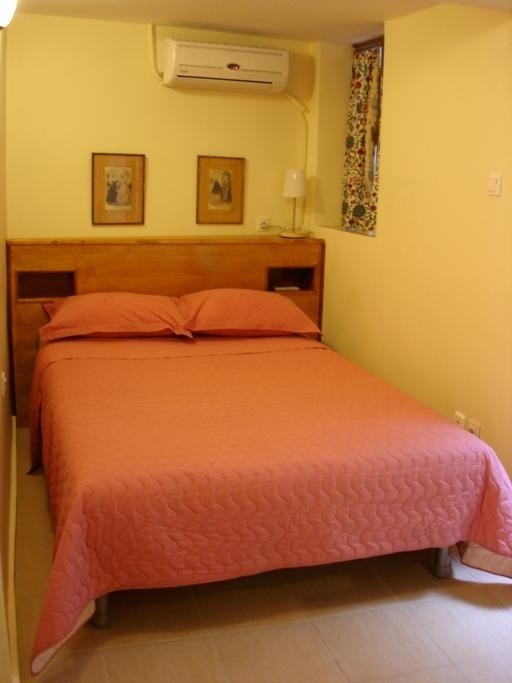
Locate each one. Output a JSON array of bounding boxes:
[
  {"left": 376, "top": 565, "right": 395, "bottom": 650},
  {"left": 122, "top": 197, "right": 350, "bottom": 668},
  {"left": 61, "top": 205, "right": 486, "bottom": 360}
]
[{"left": 7, "top": 232, "right": 493, "bottom": 675}]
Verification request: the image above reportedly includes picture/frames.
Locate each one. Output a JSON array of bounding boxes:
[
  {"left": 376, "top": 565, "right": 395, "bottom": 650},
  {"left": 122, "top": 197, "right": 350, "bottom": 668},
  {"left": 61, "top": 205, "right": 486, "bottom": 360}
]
[
  {"left": 195, "top": 155, "right": 246, "bottom": 224},
  {"left": 91, "top": 151, "right": 147, "bottom": 225}
]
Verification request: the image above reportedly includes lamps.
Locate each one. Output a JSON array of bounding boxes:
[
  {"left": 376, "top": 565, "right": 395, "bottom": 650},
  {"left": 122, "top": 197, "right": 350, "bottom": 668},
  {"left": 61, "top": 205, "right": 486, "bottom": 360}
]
[{"left": 281, "top": 169, "right": 306, "bottom": 237}]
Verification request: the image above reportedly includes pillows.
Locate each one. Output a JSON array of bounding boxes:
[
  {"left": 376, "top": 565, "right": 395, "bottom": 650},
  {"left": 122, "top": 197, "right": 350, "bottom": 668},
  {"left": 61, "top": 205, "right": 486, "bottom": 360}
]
[
  {"left": 180, "top": 289, "right": 324, "bottom": 338},
  {"left": 38, "top": 292, "right": 193, "bottom": 342}
]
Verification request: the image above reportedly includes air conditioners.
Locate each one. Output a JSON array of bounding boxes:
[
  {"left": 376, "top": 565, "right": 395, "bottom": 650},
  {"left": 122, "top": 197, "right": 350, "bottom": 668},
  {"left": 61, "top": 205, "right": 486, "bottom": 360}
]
[{"left": 161, "top": 37, "right": 291, "bottom": 93}]
